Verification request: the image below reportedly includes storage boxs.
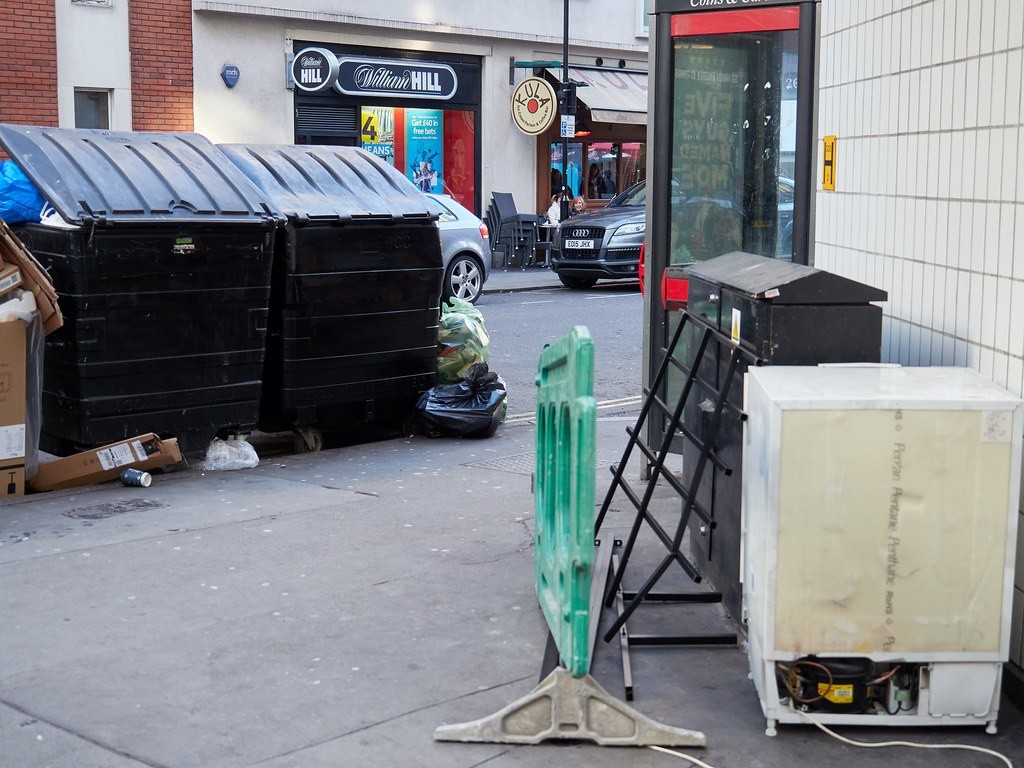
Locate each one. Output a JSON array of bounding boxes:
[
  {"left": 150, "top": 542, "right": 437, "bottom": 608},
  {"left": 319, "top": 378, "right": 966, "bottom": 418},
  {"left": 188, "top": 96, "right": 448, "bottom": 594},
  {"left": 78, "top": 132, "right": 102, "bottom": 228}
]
[{"left": 0, "top": 252, "right": 182, "bottom": 501}]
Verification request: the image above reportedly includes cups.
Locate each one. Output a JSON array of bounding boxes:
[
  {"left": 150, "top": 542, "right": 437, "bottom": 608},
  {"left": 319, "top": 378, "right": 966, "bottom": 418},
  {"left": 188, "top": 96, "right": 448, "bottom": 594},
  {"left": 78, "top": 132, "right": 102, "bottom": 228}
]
[{"left": 120, "top": 467, "right": 152, "bottom": 487}]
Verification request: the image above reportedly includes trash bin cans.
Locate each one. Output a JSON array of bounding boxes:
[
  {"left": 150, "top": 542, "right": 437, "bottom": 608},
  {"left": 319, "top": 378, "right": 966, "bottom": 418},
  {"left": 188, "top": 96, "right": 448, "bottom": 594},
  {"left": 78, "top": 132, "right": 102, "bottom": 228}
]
[
  {"left": 0, "top": 123, "right": 287, "bottom": 458},
  {"left": 218, "top": 142, "right": 442, "bottom": 457}
]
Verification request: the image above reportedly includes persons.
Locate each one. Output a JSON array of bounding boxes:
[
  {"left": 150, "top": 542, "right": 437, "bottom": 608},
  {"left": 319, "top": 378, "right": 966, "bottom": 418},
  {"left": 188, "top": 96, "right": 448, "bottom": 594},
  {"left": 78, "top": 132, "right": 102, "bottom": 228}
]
[{"left": 546, "top": 162, "right": 615, "bottom": 224}]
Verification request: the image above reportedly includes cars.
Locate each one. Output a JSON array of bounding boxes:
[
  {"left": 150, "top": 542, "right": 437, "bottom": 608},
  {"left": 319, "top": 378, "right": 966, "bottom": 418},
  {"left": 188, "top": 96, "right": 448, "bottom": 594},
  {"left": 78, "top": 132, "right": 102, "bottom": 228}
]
[
  {"left": 422, "top": 191, "right": 491, "bottom": 306},
  {"left": 550, "top": 159, "right": 795, "bottom": 288}
]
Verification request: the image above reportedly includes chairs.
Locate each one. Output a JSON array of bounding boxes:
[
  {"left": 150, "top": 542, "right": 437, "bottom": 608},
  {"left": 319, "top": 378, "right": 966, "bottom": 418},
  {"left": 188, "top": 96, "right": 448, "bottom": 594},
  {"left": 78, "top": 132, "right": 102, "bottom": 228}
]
[{"left": 483, "top": 191, "right": 552, "bottom": 270}]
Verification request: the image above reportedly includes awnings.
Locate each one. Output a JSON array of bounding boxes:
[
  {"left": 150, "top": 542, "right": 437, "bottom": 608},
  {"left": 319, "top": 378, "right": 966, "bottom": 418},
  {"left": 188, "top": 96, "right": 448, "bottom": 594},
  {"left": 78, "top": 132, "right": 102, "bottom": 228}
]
[{"left": 543, "top": 67, "right": 648, "bottom": 125}]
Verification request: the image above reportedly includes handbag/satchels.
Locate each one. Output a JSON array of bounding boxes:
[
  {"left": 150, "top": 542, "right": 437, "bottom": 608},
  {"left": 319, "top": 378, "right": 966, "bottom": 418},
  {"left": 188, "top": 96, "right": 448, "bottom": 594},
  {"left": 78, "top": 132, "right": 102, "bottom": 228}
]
[{"left": 538, "top": 212, "right": 551, "bottom": 225}]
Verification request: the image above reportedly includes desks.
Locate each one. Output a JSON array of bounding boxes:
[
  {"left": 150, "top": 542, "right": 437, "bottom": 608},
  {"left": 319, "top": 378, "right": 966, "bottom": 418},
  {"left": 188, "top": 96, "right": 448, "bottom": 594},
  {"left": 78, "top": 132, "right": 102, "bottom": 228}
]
[{"left": 539, "top": 224, "right": 560, "bottom": 268}]
